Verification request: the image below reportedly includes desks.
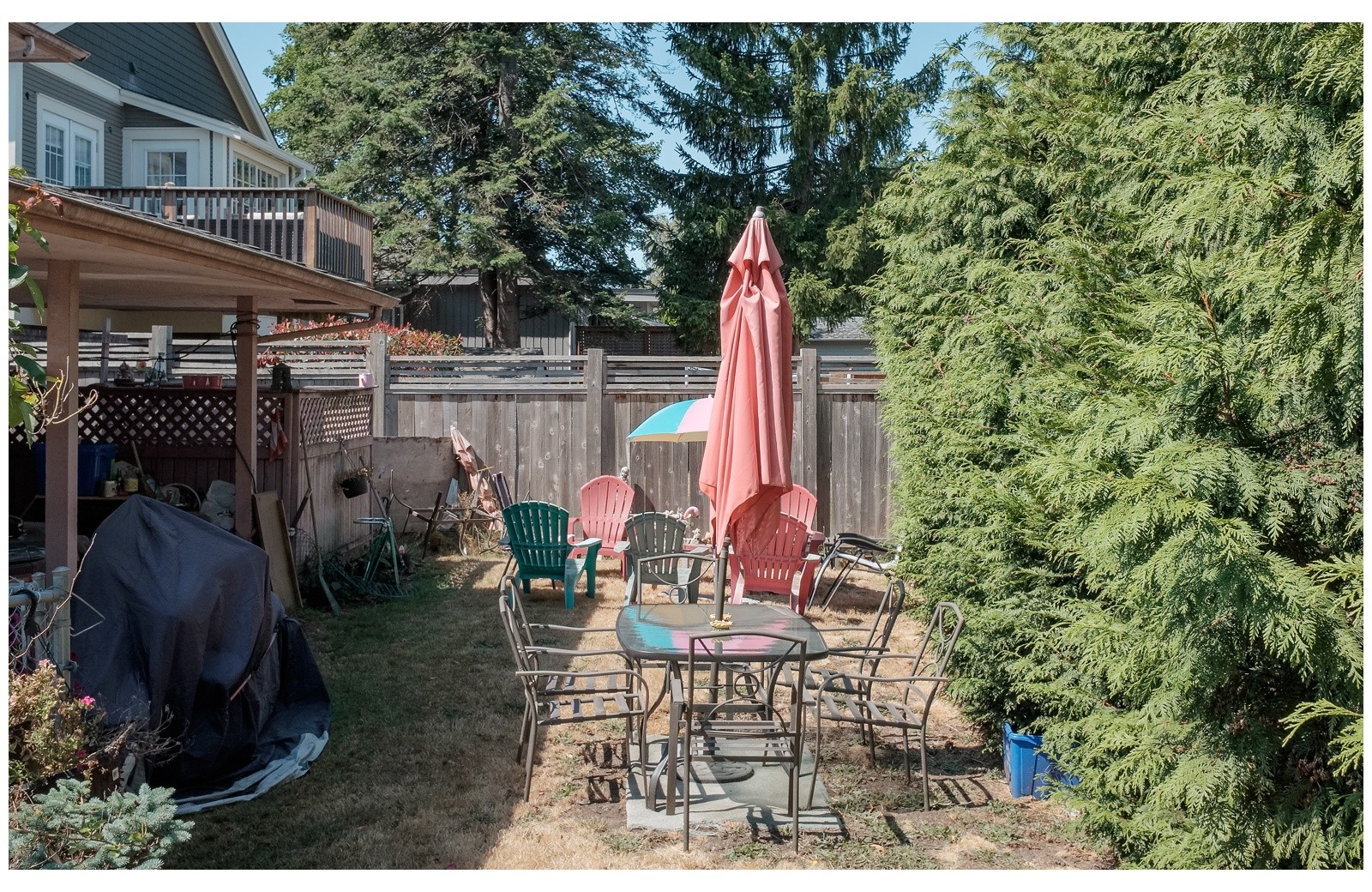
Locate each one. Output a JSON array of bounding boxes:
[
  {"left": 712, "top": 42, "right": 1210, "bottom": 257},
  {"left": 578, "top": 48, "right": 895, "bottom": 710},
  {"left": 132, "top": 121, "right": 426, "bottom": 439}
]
[
  {"left": 615, "top": 604, "right": 831, "bottom": 783},
  {"left": 34, "top": 494, "right": 164, "bottom": 529}
]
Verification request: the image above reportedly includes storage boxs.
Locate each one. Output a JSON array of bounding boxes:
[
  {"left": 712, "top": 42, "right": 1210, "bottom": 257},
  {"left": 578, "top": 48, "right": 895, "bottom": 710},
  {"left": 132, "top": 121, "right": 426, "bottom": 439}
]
[
  {"left": 35, "top": 442, "right": 118, "bottom": 494},
  {"left": 1003, "top": 723, "right": 1076, "bottom": 800}
]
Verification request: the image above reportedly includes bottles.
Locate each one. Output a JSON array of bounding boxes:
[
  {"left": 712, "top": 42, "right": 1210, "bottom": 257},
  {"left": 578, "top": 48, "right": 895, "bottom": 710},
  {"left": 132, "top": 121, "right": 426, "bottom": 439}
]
[
  {"left": 114, "top": 468, "right": 124, "bottom": 492},
  {"left": 399, "top": 544, "right": 405, "bottom": 556}
]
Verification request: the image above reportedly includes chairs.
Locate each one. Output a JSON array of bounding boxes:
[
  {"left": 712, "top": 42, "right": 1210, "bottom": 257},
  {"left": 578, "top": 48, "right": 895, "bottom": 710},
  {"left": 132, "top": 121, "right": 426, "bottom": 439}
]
[{"left": 489, "top": 469, "right": 965, "bottom": 855}]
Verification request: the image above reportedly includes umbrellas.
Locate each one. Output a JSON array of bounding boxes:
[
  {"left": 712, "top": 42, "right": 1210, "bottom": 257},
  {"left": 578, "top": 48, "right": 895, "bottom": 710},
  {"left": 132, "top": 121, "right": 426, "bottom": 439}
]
[
  {"left": 627, "top": 395, "right": 714, "bottom": 444},
  {"left": 698, "top": 204, "right": 793, "bottom": 757}
]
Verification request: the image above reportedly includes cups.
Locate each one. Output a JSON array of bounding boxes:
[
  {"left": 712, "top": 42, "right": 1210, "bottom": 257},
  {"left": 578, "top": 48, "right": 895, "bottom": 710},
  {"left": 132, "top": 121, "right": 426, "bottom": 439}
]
[
  {"left": 359, "top": 373, "right": 372, "bottom": 386},
  {"left": 182, "top": 374, "right": 223, "bottom": 389},
  {"left": 125, "top": 478, "right": 138, "bottom": 491}
]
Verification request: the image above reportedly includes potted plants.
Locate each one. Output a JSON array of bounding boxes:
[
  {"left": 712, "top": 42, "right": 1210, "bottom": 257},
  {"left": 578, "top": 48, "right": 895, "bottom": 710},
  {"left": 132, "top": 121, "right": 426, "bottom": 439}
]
[{"left": 334, "top": 466, "right": 374, "bottom": 499}]
